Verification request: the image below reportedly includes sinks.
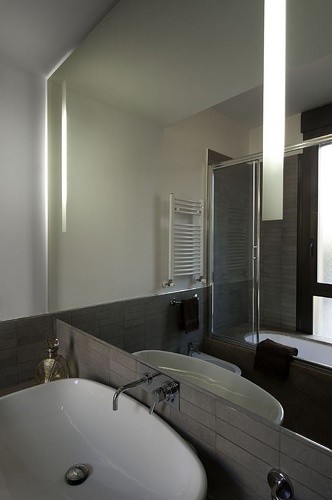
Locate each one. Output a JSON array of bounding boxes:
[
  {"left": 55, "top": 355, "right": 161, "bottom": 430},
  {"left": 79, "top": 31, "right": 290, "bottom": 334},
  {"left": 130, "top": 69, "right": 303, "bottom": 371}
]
[
  {"left": 0, "top": 378, "right": 207, "bottom": 500},
  {"left": 132, "top": 350, "right": 285, "bottom": 425}
]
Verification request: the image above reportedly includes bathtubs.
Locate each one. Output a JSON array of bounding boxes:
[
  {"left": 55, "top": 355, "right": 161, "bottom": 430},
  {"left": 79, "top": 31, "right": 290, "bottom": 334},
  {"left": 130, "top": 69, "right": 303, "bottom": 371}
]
[{"left": 242, "top": 331, "right": 332, "bottom": 373}]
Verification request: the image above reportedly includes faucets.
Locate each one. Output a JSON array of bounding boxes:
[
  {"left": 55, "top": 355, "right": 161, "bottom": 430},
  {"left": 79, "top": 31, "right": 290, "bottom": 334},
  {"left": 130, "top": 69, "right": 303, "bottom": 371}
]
[{"left": 113, "top": 372, "right": 161, "bottom": 411}]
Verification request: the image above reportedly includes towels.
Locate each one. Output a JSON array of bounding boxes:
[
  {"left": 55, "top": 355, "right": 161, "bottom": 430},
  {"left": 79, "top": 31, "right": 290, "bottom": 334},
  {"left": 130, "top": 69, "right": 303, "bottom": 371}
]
[
  {"left": 181, "top": 297, "right": 200, "bottom": 334},
  {"left": 256, "top": 338, "right": 298, "bottom": 384}
]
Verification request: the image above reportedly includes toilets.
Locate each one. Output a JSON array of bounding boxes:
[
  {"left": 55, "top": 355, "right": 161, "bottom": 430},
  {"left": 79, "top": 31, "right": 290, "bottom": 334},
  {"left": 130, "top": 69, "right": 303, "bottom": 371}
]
[{"left": 190, "top": 349, "right": 243, "bottom": 378}]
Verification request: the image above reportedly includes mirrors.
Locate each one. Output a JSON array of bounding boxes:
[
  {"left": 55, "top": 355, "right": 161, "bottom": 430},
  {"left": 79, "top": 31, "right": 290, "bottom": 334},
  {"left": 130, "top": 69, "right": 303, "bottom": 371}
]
[{"left": 46, "top": 3, "right": 332, "bottom": 451}]
